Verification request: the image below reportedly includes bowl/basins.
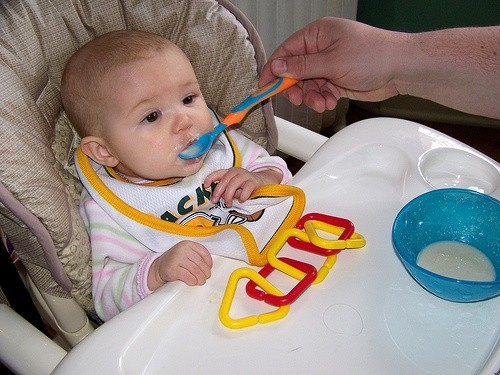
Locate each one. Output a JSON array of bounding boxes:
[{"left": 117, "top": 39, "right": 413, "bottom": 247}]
[{"left": 392, "top": 187, "right": 500, "bottom": 303}]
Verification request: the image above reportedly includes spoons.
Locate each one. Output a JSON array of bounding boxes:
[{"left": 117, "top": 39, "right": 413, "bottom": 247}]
[{"left": 178, "top": 77, "right": 299, "bottom": 159}]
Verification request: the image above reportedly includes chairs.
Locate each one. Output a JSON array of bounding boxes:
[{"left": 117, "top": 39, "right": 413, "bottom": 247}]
[{"left": 0, "top": 0, "right": 329, "bottom": 375}]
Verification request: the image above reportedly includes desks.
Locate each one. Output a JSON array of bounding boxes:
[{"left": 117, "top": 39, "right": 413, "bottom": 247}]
[{"left": 50, "top": 117, "right": 500, "bottom": 375}]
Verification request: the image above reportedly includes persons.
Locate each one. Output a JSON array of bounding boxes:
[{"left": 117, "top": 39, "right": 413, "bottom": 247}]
[
  {"left": 257, "top": 16, "right": 500, "bottom": 119},
  {"left": 60, "top": 29, "right": 293, "bottom": 323}
]
